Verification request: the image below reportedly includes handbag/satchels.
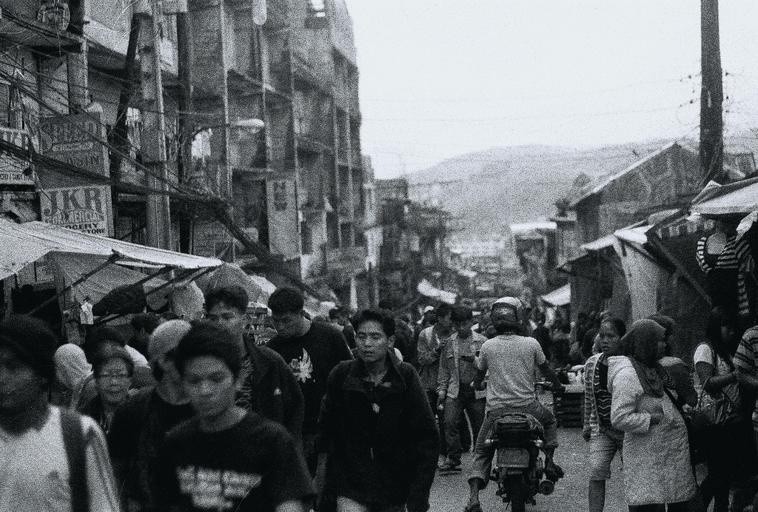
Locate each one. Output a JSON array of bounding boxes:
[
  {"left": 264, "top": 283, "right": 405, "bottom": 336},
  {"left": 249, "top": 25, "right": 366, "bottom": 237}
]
[
  {"left": 694, "top": 376, "right": 747, "bottom": 438},
  {"left": 680, "top": 406, "right": 706, "bottom": 447}
]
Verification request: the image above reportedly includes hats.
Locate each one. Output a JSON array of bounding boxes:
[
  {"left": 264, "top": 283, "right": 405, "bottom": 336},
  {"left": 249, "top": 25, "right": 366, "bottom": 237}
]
[
  {"left": 0, "top": 313, "right": 59, "bottom": 383},
  {"left": 146, "top": 319, "right": 193, "bottom": 365}
]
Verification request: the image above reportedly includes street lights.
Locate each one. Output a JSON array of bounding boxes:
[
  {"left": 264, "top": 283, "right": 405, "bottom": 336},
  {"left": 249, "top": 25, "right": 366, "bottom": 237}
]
[{"left": 168, "top": 115, "right": 271, "bottom": 259}]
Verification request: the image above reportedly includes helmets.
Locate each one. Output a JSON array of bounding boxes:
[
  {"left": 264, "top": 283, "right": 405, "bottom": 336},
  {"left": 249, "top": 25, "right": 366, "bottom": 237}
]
[{"left": 489, "top": 296, "right": 527, "bottom": 334}]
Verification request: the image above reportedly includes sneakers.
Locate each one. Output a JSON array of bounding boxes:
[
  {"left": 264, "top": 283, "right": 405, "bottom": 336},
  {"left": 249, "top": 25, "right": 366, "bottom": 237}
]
[{"left": 439, "top": 461, "right": 463, "bottom": 476}]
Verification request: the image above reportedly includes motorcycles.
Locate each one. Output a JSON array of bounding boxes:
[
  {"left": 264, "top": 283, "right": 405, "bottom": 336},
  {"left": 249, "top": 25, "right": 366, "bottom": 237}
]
[{"left": 467, "top": 374, "right": 565, "bottom": 510}]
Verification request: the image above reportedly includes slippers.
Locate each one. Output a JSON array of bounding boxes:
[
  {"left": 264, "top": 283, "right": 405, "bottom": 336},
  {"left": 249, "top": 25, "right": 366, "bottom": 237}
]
[
  {"left": 464, "top": 501, "right": 482, "bottom": 512},
  {"left": 545, "top": 463, "right": 565, "bottom": 479}
]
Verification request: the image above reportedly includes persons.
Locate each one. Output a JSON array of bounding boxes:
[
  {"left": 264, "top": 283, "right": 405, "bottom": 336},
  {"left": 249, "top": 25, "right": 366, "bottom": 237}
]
[
  {"left": 2, "top": 283, "right": 758, "bottom": 512},
  {"left": 695, "top": 217, "right": 756, "bottom": 355}
]
